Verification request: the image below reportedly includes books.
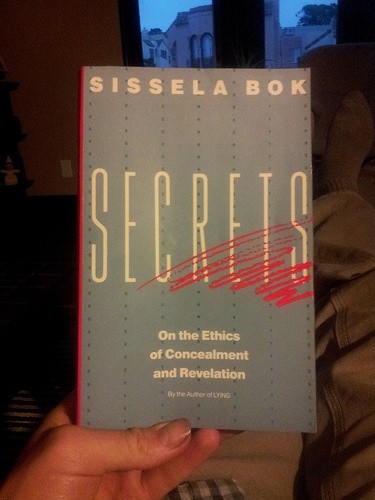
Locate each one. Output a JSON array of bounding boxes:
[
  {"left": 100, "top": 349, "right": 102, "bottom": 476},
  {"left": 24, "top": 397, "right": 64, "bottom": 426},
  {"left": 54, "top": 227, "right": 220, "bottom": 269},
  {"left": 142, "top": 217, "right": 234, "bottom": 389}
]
[{"left": 78, "top": 68, "right": 318, "bottom": 435}]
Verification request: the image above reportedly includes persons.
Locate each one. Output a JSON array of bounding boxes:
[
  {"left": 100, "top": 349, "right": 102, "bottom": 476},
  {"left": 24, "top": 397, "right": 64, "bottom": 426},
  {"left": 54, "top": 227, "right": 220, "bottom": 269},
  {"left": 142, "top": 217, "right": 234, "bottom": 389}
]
[{"left": 0, "top": 91, "right": 375, "bottom": 500}]
